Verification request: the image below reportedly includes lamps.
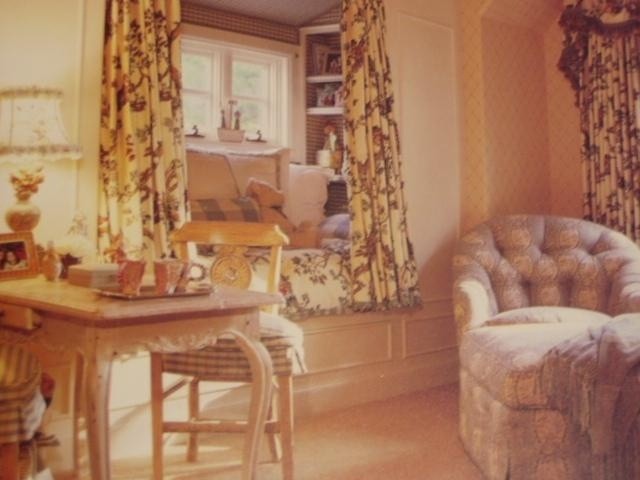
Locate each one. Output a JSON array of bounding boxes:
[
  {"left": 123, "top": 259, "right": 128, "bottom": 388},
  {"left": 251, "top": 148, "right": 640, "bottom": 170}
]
[{"left": 0, "top": 87, "right": 83, "bottom": 231}]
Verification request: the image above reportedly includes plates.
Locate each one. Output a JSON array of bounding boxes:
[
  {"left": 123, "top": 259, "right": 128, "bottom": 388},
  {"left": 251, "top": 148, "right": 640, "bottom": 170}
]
[{"left": 91, "top": 278, "right": 216, "bottom": 303}]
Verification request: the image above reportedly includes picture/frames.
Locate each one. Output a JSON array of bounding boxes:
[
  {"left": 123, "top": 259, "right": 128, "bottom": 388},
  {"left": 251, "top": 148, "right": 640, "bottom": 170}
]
[
  {"left": 0, "top": 231, "right": 37, "bottom": 281},
  {"left": 321, "top": 52, "right": 342, "bottom": 74}
]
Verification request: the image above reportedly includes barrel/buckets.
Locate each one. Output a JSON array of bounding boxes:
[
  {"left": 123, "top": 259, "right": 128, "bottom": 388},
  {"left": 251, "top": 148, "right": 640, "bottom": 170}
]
[{"left": 317, "top": 149, "right": 330, "bottom": 167}]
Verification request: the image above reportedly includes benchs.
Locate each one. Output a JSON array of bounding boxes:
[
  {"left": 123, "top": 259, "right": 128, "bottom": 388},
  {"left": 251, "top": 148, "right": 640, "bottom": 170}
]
[{"left": 182, "top": 239, "right": 352, "bottom": 318}]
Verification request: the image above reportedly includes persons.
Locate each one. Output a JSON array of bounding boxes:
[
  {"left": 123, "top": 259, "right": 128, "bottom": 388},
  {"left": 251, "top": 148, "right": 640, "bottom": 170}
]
[
  {"left": 0, "top": 246, "right": 7, "bottom": 270},
  {"left": 5, "top": 251, "right": 26, "bottom": 270}
]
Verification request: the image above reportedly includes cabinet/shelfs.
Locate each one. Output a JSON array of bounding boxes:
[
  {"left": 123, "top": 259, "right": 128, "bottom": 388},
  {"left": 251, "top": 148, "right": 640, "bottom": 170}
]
[{"left": 298, "top": 23, "right": 346, "bottom": 183}]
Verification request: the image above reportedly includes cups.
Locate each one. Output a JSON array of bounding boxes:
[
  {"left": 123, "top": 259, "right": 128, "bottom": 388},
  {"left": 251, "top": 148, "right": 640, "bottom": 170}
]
[
  {"left": 152, "top": 255, "right": 208, "bottom": 298},
  {"left": 115, "top": 256, "right": 147, "bottom": 297}
]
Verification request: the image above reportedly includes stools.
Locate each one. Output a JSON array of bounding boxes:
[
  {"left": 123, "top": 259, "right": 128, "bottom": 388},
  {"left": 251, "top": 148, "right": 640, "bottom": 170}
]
[{"left": 459, "top": 326, "right": 594, "bottom": 480}]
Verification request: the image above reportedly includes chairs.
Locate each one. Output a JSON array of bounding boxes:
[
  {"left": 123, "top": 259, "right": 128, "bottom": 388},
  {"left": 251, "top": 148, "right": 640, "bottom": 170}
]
[
  {"left": 450, "top": 212, "right": 640, "bottom": 350},
  {"left": 539, "top": 308, "right": 640, "bottom": 480},
  {"left": 0, "top": 341, "right": 47, "bottom": 480},
  {"left": 148, "top": 219, "right": 307, "bottom": 480}
]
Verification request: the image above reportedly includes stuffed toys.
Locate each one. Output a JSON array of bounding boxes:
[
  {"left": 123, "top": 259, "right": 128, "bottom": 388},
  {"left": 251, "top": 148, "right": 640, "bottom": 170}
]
[
  {"left": 281, "top": 168, "right": 329, "bottom": 225},
  {"left": 245, "top": 176, "right": 317, "bottom": 247}
]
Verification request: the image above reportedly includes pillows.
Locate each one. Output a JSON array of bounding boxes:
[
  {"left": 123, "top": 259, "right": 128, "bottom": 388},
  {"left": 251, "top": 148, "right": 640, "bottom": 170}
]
[{"left": 184, "top": 146, "right": 350, "bottom": 247}]
